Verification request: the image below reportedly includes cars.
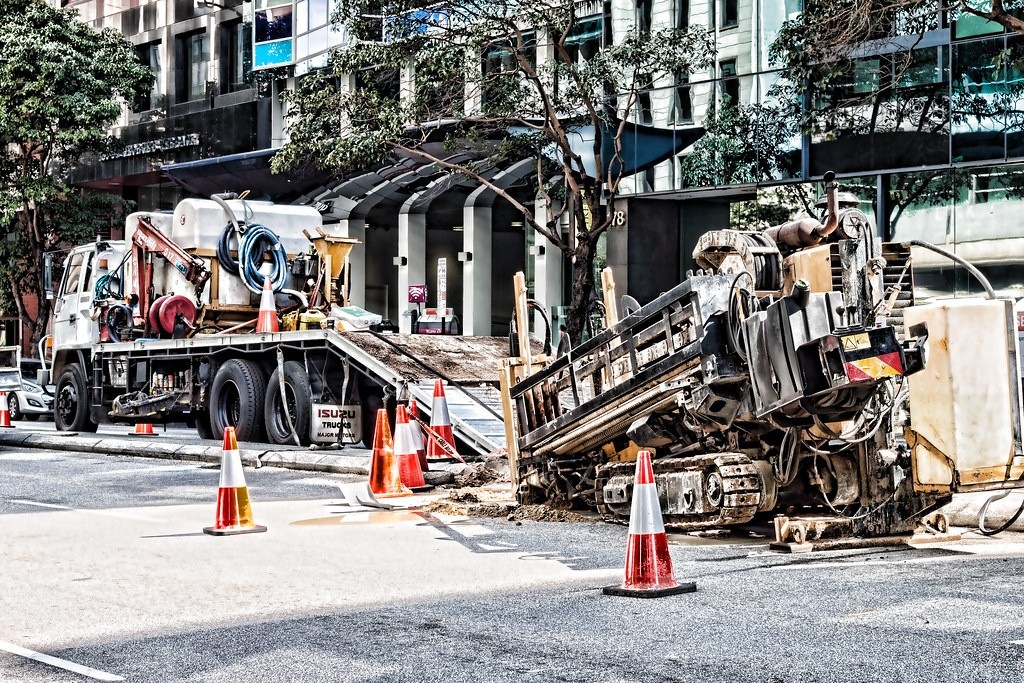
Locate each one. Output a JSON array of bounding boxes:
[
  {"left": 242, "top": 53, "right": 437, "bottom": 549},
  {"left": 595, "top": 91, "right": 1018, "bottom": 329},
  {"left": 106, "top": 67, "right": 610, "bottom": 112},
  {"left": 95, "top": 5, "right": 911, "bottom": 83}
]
[{"left": 0, "top": 357, "right": 57, "bottom": 422}]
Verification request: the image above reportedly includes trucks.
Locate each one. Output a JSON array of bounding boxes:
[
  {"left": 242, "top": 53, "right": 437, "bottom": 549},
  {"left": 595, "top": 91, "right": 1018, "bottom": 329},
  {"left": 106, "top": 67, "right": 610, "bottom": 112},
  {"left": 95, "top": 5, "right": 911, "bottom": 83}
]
[{"left": 42, "top": 239, "right": 584, "bottom": 462}]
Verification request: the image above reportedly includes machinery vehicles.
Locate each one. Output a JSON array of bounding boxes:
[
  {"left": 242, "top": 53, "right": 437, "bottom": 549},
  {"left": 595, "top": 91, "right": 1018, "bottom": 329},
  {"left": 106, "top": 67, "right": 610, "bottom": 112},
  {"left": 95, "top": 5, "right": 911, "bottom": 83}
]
[{"left": 508, "top": 168, "right": 1024, "bottom": 555}]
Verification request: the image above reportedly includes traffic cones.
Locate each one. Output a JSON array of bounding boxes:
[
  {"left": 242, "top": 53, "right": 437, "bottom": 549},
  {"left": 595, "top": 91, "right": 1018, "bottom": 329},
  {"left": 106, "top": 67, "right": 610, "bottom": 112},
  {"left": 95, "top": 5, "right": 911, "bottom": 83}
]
[
  {"left": 129, "top": 423, "right": 160, "bottom": 437},
  {"left": 422, "top": 377, "right": 466, "bottom": 464},
  {"left": 370, "top": 408, "right": 416, "bottom": 500},
  {"left": 603, "top": 450, "right": 697, "bottom": 599},
  {"left": 0, "top": 391, "right": 16, "bottom": 428},
  {"left": 203, "top": 426, "right": 267, "bottom": 536},
  {"left": 406, "top": 398, "right": 433, "bottom": 474},
  {"left": 392, "top": 405, "right": 436, "bottom": 493},
  {"left": 253, "top": 274, "right": 279, "bottom": 333}
]
